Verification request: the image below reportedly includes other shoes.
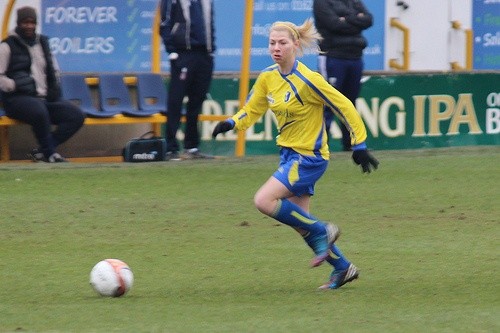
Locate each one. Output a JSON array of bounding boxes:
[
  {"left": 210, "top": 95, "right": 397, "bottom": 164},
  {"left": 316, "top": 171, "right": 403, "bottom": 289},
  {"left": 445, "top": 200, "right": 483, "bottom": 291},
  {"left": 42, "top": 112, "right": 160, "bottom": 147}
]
[
  {"left": 31, "top": 151, "right": 43, "bottom": 162},
  {"left": 47, "top": 153, "right": 66, "bottom": 164}
]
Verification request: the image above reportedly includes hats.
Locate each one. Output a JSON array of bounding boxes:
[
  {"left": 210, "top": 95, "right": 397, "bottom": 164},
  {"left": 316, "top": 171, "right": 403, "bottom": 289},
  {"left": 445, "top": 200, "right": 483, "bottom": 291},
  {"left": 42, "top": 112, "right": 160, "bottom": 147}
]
[{"left": 17, "top": 7, "right": 36, "bottom": 23}]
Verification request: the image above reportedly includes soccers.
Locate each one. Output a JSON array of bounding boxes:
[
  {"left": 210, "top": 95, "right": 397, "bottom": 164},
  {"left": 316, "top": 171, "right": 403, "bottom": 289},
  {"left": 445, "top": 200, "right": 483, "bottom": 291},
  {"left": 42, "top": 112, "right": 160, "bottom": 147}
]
[{"left": 89, "top": 259, "right": 134, "bottom": 298}]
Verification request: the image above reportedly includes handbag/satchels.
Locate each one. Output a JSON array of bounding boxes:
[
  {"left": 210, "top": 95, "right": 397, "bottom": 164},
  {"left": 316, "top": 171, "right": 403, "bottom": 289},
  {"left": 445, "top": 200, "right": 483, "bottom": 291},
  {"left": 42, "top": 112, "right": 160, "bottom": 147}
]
[{"left": 121, "top": 131, "right": 172, "bottom": 162}]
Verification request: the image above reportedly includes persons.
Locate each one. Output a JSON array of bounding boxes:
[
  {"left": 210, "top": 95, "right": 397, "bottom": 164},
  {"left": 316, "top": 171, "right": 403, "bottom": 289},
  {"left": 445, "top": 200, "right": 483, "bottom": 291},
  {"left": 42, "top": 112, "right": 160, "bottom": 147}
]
[
  {"left": 211, "top": 20, "right": 381, "bottom": 291},
  {"left": 312, "top": 0, "right": 374, "bottom": 153},
  {"left": 157, "top": 0, "right": 216, "bottom": 162},
  {"left": 0, "top": 6, "right": 87, "bottom": 164}
]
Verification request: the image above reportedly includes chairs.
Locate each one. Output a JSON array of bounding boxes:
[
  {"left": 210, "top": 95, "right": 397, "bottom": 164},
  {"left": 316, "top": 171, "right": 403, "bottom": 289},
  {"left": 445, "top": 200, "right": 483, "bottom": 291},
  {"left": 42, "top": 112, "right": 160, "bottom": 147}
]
[
  {"left": 136, "top": 73, "right": 190, "bottom": 116},
  {"left": 98, "top": 73, "right": 156, "bottom": 117},
  {"left": 60, "top": 74, "right": 122, "bottom": 119}
]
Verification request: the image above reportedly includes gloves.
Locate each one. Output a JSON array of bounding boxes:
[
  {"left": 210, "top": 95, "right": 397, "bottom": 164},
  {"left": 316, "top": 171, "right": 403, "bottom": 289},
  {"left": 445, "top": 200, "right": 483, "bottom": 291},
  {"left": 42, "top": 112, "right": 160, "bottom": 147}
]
[
  {"left": 211, "top": 120, "right": 235, "bottom": 138},
  {"left": 352, "top": 144, "right": 380, "bottom": 174}
]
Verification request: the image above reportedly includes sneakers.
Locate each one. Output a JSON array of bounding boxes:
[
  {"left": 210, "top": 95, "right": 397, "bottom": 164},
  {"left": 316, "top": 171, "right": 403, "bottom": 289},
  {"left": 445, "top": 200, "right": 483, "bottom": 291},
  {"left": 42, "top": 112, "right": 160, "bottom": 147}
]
[
  {"left": 316, "top": 262, "right": 360, "bottom": 289},
  {"left": 308, "top": 220, "right": 339, "bottom": 267}
]
